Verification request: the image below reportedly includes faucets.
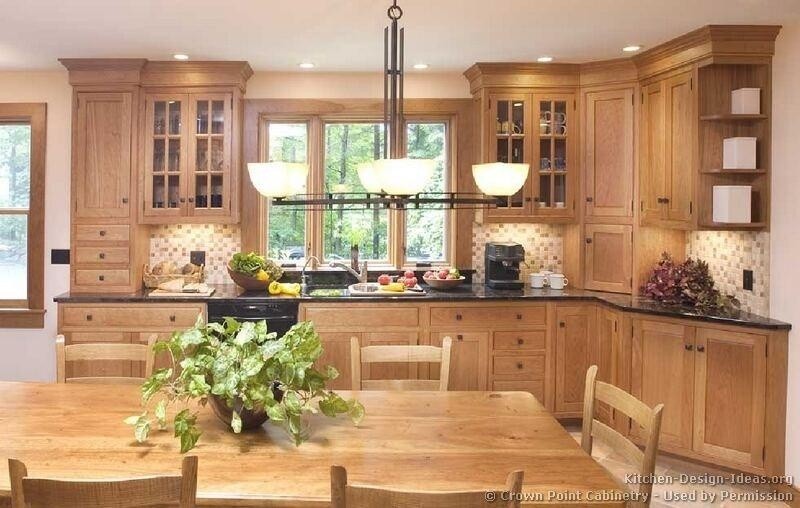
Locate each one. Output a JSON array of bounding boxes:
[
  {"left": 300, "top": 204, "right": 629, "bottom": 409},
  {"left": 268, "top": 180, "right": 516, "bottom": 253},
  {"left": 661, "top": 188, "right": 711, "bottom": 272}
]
[
  {"left": 300, "top": 256, "right": 320, "bottom": 294},
  {"left": 328, "top": 261, "right": 367, "bottom": 283}
]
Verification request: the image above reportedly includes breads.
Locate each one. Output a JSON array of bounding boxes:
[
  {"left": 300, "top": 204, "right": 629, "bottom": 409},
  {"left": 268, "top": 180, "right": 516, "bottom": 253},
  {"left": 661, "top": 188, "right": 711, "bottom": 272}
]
[{"left": 153, "top": 262, "right": 199, "bottom": 275}]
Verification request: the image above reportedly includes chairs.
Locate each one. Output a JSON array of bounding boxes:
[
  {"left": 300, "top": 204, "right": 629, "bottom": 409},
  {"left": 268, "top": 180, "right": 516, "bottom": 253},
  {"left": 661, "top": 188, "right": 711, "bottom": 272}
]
[
  {"left": 7, "top": 454, "right": 199, "bottom": 507},
  {"left": 579, "top": 364, "right": 665, "bottom": 507},
  {"left": 54, "top": 334, "right": 160, "bottom": 385},
  {"left": 329, "top": 463, "right": 524, "bottom": 508},
  {"left": 349, "top": 335, "right": 452, "bottom": 391}
]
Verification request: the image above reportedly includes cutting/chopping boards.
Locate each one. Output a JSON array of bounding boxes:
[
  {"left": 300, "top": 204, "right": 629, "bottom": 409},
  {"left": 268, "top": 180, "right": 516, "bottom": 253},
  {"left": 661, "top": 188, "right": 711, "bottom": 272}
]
[{"left": 348, "top": 281, "right": 426, "bottom": 296}]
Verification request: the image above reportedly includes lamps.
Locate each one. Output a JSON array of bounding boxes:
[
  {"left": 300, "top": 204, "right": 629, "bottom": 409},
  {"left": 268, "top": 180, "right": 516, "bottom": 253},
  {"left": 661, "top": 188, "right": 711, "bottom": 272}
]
[{"left": 246, "top": 0, "right": 528, "bottom": 213}]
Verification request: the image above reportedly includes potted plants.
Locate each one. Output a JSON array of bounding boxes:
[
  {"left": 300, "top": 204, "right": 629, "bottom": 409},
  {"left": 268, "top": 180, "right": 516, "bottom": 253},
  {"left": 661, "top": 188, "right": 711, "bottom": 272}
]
[{"left": 126, "top": 313, "right": 366, "bottom": 457}]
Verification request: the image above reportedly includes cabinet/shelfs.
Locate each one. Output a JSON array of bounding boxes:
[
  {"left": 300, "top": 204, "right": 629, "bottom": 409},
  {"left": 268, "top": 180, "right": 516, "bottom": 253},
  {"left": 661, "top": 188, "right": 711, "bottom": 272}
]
[
  {"left": 563, "top": 223, "right": 685, "bottom": 299},
  {"left": 551, "top": 297, "right": 623, "bottom": 430},
  {"left": 59, "top": 57, "right": 148, "bottom": 222},
  {"left": 423, "top": 301, "right": 551, "bottom": 414},
  {"left": 298, "top": 302, "right": 424, "bottom": 390},
  {"left": 620, "top": 310, "right": 790, "bottom": 496},
  {"left": 582, "top": 53, "right": 639, "bottom": 225},
  {"left": 140, "top": 59, "right": 254, "bottom": 227},
  {"left": 463, "top": 61, "right": 580, "bottom": 225},
  {"left": 696, "top": 22, "right": 785, "bottom": 233},
  {"left": 57, "top": 303, "right": 208, "bottom": 384},
  {"left": 68, "top": 219, "right": 150, "bottom": 295},
  {"left": 632, "top": 26, "right": 697, "bottom": 232}
]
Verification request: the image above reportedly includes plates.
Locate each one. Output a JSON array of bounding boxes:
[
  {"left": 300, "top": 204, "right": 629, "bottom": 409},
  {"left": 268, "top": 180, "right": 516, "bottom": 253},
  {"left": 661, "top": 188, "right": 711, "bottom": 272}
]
[
  {"left": 156, "top": 202, "right": 175, "bottom": 209},
  {"left": 198, "top": 194, "right": 222, "bottom": 207}
]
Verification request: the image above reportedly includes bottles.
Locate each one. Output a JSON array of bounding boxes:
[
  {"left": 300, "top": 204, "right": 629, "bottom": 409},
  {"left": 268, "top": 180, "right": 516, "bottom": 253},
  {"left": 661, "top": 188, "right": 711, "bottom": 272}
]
[{"left": 512, "top": 144, "right": 520, "bottom": 162}]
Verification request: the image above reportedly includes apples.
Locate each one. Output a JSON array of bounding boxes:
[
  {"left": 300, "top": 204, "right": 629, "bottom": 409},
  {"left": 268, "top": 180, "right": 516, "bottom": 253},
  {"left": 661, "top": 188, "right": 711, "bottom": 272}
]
[
  {"left": 397, "top": 271, "right": 417, "bottom": 287},
  {"left": 424, "top": 268, "right": 460, "bottom": 279},
  {"left": 378, "top": 274, "right": 391, "bottom": 285}
]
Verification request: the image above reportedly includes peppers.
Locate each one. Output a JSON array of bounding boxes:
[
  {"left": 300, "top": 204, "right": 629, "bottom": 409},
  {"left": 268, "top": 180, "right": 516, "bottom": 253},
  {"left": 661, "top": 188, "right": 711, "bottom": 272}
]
[
  {"left": 381, "top": 282, "right": 411, "bottom": 291},
  {"left": 255, "top": 271, "right": 300, "bottom": 294}
]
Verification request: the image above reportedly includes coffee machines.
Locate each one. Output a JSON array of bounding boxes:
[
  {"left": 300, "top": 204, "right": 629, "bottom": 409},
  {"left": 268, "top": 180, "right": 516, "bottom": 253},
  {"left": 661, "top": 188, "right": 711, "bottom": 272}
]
[{"left": 483, "top": 240, "right": 526, "bottom": 289}]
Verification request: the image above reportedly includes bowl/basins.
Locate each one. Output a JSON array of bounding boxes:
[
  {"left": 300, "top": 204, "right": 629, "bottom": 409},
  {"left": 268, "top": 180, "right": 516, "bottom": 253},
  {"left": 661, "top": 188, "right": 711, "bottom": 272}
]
[
  {"left": 229, "top": 267, "right": 283, "bottom": 290},
  {"left": 553, "top": 202, "right": 564, "bottom": 209},
  {"left": 423, "top": 276, "right": 466, "bottom": 289},
  {"left": 539, "top": 201, "right": 546, "bottom": 206}
]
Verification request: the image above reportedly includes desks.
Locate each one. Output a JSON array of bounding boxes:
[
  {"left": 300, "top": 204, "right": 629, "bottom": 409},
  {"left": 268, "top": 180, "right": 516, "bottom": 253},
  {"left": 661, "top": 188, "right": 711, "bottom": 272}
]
[{"left": 1, "top": 379, "right": 629, "bottom": 506}]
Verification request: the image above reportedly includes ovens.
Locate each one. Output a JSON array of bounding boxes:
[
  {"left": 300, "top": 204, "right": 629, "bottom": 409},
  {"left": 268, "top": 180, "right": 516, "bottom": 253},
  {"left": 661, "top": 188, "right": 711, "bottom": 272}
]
[{"left": 205, "top": 301, "right": 298, "bottom": 342}]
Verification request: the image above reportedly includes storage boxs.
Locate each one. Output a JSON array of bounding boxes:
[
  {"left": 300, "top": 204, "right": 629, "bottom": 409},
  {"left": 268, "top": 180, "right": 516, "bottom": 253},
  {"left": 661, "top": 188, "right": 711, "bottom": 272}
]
[
  {"left": 722, "top": 135, "right": 757, "bottom": 169},
  {"left": 712, "top": 184, "right": 753, "bottom": 223},
  {"left": 730, "top": 87, "right": 761, "bottom": 114}
]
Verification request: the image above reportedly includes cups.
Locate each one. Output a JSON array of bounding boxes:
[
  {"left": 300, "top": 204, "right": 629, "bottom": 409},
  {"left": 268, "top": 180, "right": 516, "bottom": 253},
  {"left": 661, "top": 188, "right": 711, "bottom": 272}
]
[
  {"left": 530, "top": 273, "right": 549, "bottom": 288},
  {"left": 541, "top": 156, "right": 566, "bottom": 171},
  {"left": 497, "top": 107, "right": 567, "bottom": 136},
  {"left": 540, "top": 271, "right": 553, "bottom": 286},
  {"left": 550, "top": 273, "right": 568, "bottom": 289},
  {"left": 155, "top": 113, "right": 207, "bottom": 134}
]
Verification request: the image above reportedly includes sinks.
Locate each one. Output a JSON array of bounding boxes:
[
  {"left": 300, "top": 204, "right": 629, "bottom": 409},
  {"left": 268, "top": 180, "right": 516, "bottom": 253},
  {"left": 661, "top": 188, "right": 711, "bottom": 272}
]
[
  {"left": 348, "top": 283, "right": 379, "bottom": 297},
  {"left": 303, "top": 284, "right": 350, "bottom": 297}
]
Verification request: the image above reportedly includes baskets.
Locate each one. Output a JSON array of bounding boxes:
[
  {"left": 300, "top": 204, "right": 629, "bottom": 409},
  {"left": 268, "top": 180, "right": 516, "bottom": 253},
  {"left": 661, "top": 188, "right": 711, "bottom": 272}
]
[{"left": 141, "top": 261, "right": 204, "bottom": 287}]
